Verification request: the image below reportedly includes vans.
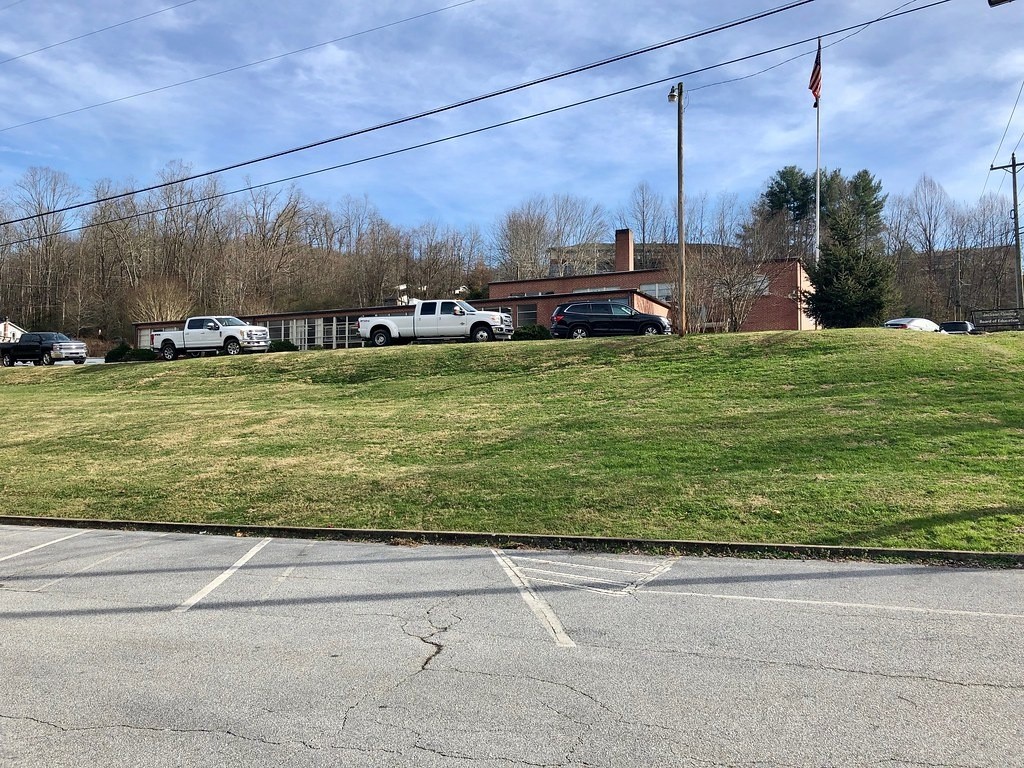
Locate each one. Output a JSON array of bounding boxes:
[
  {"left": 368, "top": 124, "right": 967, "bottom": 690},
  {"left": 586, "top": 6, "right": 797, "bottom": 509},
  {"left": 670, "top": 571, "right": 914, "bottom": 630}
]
[{"left": 935, "top": 318, "right": 975, "bottom": 335}]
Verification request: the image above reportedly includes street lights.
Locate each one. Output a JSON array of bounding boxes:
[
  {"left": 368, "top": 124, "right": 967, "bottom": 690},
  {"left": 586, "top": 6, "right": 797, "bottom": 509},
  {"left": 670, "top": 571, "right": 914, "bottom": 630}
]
[{"left": 667, "top": 82, "right": 688, "bottom": 337}]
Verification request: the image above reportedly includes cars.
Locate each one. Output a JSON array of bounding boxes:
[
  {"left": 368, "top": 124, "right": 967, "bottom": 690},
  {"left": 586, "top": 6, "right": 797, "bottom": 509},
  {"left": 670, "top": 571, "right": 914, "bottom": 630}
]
[{"left": 883, "top": 317, "right": 949, "bottom": 334}]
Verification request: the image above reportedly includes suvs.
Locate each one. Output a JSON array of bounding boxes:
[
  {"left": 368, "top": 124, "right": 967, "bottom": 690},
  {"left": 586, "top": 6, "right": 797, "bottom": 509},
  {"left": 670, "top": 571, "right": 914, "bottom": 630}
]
[{"left": 549, "top": 300, "right": 671, "bottom": 339}]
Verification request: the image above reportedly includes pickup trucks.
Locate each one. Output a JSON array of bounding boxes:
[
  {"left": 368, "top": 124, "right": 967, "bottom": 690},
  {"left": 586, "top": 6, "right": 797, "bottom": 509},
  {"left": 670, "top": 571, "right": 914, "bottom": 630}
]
[
  {"left": 0, "top": 332, "right": 88, "bottom": 367},
  {"left": 356, "top": 298, "right": 515, "bottom": 346},
  {"left": 149, "top": 316, "right": 271, "bottom": 361}
]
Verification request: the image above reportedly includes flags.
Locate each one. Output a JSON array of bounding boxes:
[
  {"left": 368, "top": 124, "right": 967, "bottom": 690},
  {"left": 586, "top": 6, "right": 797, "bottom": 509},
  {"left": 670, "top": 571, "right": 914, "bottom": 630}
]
[{"left": 809, "top": 40, "right": 821, "bottom": 108}]
